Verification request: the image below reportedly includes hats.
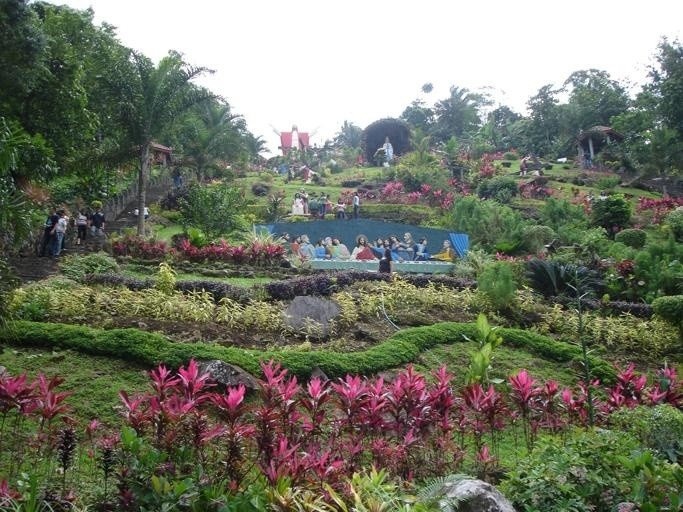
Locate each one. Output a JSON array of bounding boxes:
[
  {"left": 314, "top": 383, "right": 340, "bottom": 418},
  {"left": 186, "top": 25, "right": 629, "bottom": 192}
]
[
  {"left": 356, "top": 234, "right": 369, "bottom": 245},
  {"left": 290, "top": 233, "right": 300, "bottom": 242}
]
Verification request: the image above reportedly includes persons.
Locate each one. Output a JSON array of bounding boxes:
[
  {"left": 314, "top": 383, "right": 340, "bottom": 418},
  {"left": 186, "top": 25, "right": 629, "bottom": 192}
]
[
  {"left": 143, "top": 205, "right": 150, "bottom": 218},
  {"left": 516, "top": 152, "right": 531, "bottom": 175},
  {"left": 59, "top": 211, "right": 69, "bottom": 251},
  {"left": 36, "top": 203, "right": 58, "bottom": 258},
  {"left": 49, "top": 210, "right": 67, "bottom": 260},
  {"left": 73, "top": 207, "right": 88, "bottom": 246},
  {"left": 376, "top": 248, "right": 394, "bottom": 274},
  {"left": 287, "top": 186, "right": 360, "bottom": 220},
  {"left": 132, "top": 207, "right": 139, "bottom": 218},
  {"left": 376, "top": 134, "right": 393, "bottom": 167},
  {"left": 280, "top": 230, "right": 458, "bottom": 262},
  {"left": 89, "top": 206, "right": 105, "bottom": 235},
  {"left": 303, "top": 163, "right": 318, "bottom": 185}
]
